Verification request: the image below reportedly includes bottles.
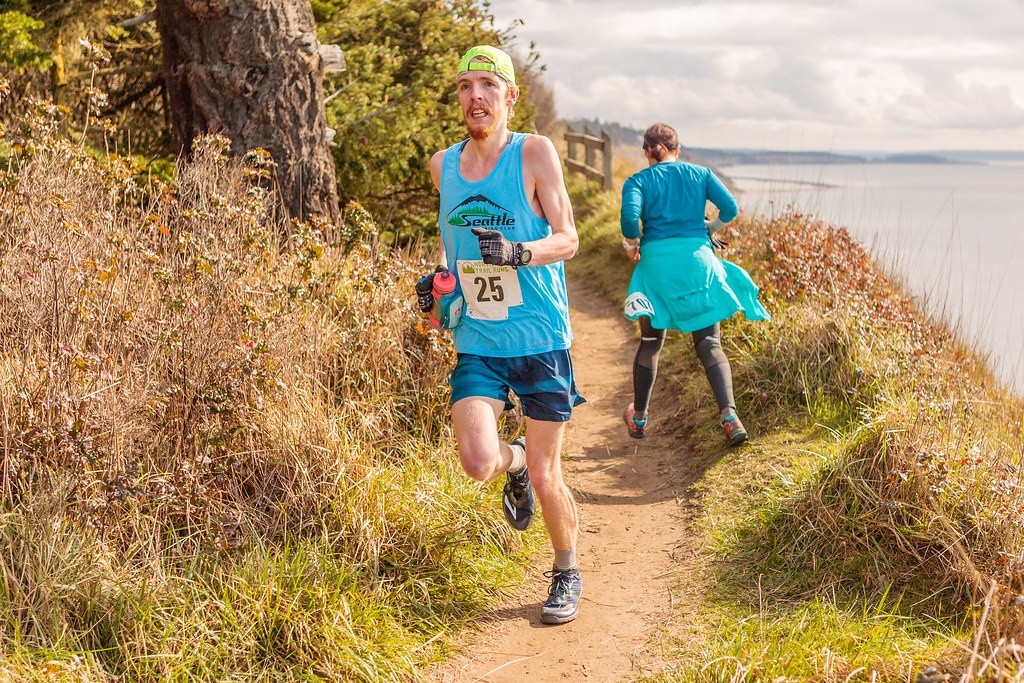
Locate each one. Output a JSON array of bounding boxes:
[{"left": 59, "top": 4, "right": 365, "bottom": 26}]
[{"left": 427, "top": 271, "right": 456, "bottom": 327}]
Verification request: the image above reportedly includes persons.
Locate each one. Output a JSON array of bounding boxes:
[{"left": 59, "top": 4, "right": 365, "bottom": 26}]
[
  {"left": 620, "top": 123, "right": 770, "bottom": 444},
  {"left": 415, "top": 44, "right": 586, "bottom": 623}
]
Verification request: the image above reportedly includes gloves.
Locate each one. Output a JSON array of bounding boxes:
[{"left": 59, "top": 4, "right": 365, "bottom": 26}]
[
  {"left": 705, "top": 217, "right": 729, "bottom": 249},
  {"left": 471, "top": 228, "right": 521, "bottom": 269},
  {"left": 415, "top": 265, "right": 449, "bottom": 312}
]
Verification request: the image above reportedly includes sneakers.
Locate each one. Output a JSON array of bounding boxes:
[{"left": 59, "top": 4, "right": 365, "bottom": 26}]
[
  {"left": 624, "top": 401, "right": 647, "bottom": 439},
  {"left": 540, "top": 563, "right": 583, "bottom": 623},
  {"left": 720, "top": 413, "right": 749, "bottom": 445},
  {"left": 502, "top": 436, "right": 537, "bottom": 531}
]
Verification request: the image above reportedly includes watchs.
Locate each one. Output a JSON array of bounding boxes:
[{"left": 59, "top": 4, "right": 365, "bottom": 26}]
[
  {"left": 623, "top": 238, "right": 639, "bottom": 250},
  {"left": 517, "top": 242, "right": 532, "bottom": 266}
]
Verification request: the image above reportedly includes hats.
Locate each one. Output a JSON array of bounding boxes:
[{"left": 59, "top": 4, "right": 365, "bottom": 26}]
[{"left": 457, "top": 45, "right": 515, "bottom": 85}]
